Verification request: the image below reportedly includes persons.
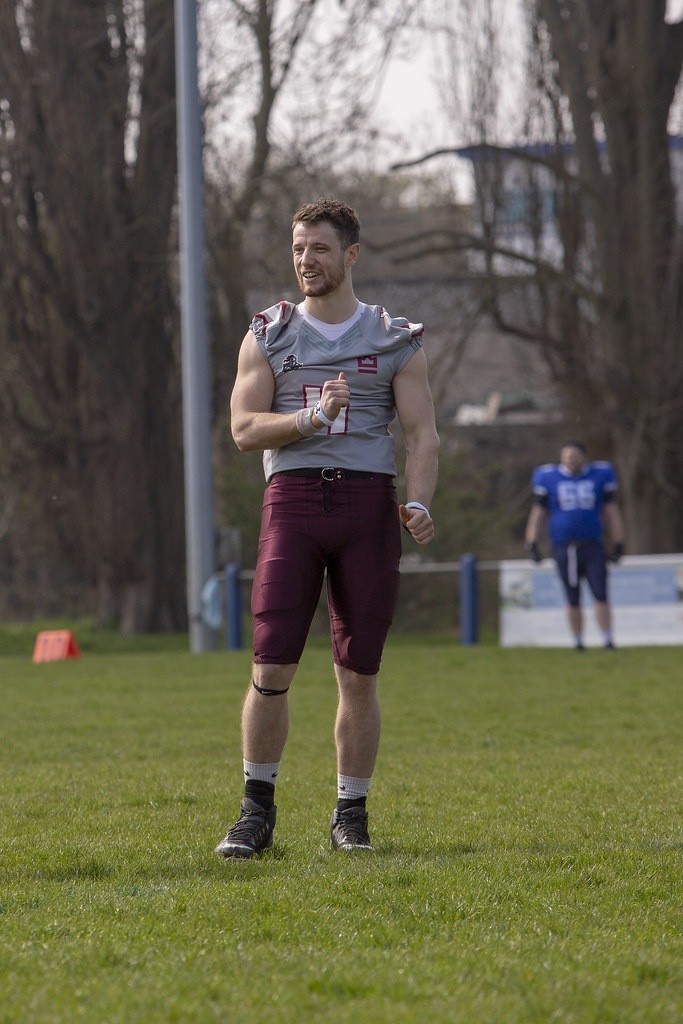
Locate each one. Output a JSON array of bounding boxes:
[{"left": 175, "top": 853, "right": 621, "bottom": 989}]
[
  {"left": 520, "top": 440, "right": 628, "bottom": 651},
  {"left": 209, "top": 198, "right": 444, "bottom": 862}
]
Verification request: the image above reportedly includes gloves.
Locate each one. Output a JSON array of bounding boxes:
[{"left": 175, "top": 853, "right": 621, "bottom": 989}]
[
  {"left": 526, "top": 544, "right": 541, "bottom": 563},
  {"left": 610, "top": 544, "right": 623, "bottom": 562}
]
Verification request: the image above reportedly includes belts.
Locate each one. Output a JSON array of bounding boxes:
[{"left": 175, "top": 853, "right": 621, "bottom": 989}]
[{"left": 278, "top": 468, "right": 391, "bottom": 481}]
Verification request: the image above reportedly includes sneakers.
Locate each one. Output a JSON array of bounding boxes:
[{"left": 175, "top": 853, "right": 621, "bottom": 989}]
[
  {"left": 329, "top": 806, "right": 375, "bottom": 853},
  {"left": 215, "top": 797, "right": 277, "bottom": 858}
]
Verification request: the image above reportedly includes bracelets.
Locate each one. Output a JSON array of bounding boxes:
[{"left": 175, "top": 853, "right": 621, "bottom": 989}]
[
  {"left": 398, "top": 500, "right": 433, "bottom": 535},
  {"left": 312, "top": 401, "right": 335, "bottom": 428}
]
